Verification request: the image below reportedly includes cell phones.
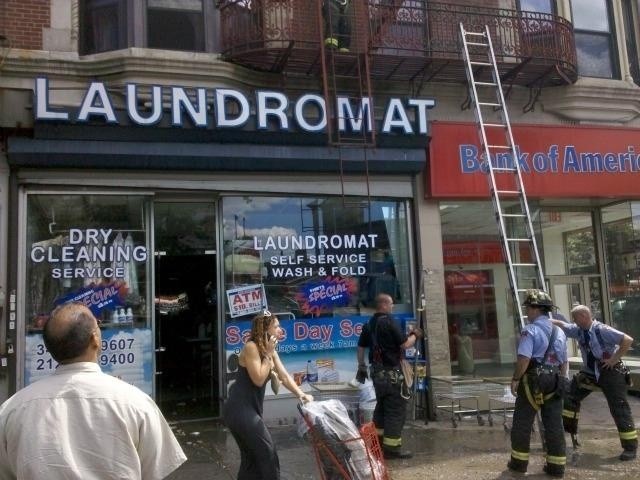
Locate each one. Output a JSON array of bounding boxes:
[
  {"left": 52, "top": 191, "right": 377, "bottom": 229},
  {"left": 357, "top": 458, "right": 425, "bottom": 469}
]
[{"left": 265, "top": 333, "right": 272, "bottom": 343}]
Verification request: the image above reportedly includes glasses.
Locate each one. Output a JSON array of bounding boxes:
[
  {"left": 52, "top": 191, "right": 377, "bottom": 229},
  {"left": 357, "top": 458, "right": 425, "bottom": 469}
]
[{"left": 91, "top": 319, "right": 101, "bottom": 337}]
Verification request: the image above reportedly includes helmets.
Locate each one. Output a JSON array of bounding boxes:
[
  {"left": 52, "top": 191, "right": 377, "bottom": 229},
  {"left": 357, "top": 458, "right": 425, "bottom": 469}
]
[{"left": 521, "top": 290, "right": 553, "bottom": 312}]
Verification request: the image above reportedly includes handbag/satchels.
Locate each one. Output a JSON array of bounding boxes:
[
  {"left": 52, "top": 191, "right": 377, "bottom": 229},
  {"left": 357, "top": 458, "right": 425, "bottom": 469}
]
[{"left": 270, "top": 370, "right": 279, "bottom": 395}]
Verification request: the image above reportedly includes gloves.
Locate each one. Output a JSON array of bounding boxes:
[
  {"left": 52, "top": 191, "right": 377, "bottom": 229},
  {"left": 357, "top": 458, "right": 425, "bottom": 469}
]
[
  {"left": 410, "top": 327, "right": 424, "bottom": 338},
  {"left": 356, "top": 364, "right": 368, "bottom": 384}
]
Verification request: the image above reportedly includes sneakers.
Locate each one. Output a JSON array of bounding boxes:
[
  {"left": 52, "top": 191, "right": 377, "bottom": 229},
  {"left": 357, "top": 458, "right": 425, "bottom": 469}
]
[
  {"left": 543, "top": 465, "right": 564, "bottom": 477},
  {"left": 383, "top": 450, "right": 413, "bottom": 460},
  {"left": 620, "top": 450, "right": 636, "bottom": 461},
  {"left": 507, "top": 460, "right": 527, "bottom": 472}
]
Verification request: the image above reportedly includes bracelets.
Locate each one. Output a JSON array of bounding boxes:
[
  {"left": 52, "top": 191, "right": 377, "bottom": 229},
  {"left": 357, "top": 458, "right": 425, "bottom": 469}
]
[{"left": 512, "top": 377, "right": 519, "bottom": 381}]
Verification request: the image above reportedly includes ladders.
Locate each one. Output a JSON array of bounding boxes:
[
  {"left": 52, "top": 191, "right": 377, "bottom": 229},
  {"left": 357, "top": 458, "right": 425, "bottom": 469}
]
[{"left": 458, "top": 22, "right": 578, "bottom": 451}]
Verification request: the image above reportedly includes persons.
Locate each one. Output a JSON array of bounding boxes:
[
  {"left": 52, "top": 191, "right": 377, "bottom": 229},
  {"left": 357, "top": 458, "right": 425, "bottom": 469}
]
[
  {"left": 322, "top": 0, "right": 351, "bottom": 53},
  {"left": 507, "top": 290, "right": 570, "bottom": 477},
  {"left": 548, "top": 304, "right": 638, "bottom": 460},
  {"left": 225, "top": 313, "right": 314, "bottom": 479},
  {"left": 572, "top": 295, "right": 579, "bottom": 307},
  {"left": 358, "top": 294, "right": 423, "bottom": 460},
  {"left": 0, "top": 301, "right": 188, "bottom": 480}
]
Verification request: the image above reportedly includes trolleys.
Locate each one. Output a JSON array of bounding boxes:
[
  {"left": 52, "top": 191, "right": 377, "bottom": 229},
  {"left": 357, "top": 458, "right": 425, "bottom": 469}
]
[{"left": 296, "top": 396, "right": 391, "bottom": 480}]
